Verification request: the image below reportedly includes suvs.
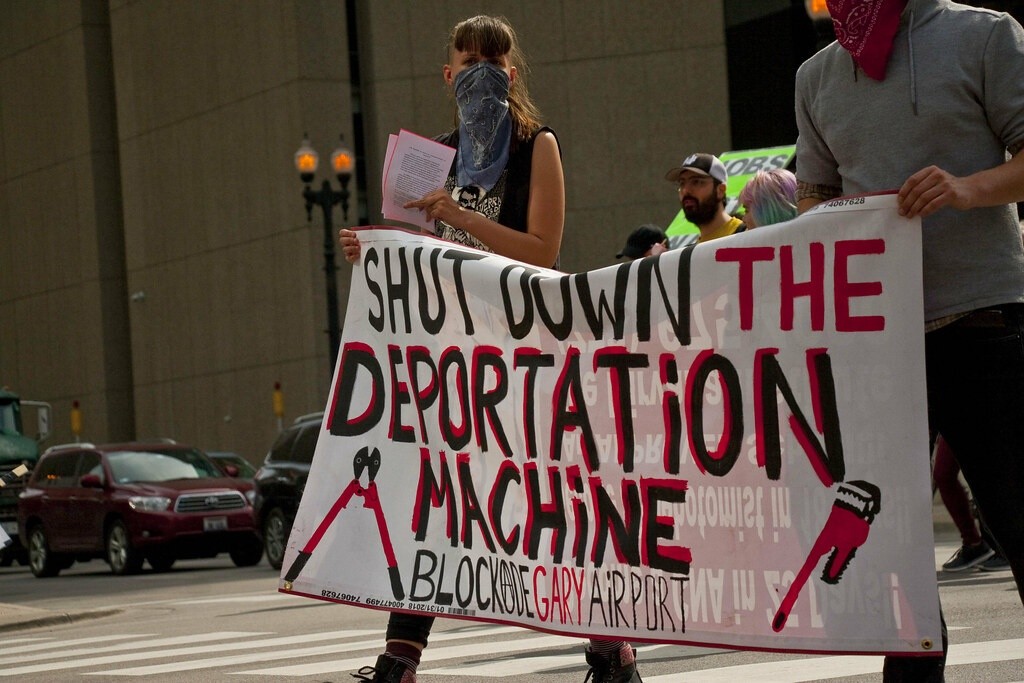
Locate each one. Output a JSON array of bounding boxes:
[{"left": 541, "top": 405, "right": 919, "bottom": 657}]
[
  {"left": 17, "top": 437, "right": 264, "bottom": 577},
  {"left": 252, "top": 413, "right": 326, "bottom": 569}
]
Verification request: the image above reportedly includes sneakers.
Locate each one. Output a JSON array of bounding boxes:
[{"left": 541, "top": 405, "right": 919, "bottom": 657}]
[
  {"left": 583, "top": 643, "right": 642, "bottom": 683},
  {"left": 979, "top": 552, "right": 1012, "bottom": 570},
  {"left": 942, "top": 539, "right": 995, "bottom": 572},
  {"left": 350, "top": 654, "right": 416, "bottom": 683}
]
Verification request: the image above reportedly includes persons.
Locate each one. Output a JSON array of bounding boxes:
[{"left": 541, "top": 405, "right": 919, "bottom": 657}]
[
  {"left": 663, "top": 152, "right": 748, "bottom": 243},
  {"left": 793, "top": 0, "right": 1024, "bottom": 683},
  {"left": 614, "top": 223, "right": 669, "bottom": 260},
  {"left": 339, "top": 14, "right": 642, "bottom": 683},
  {"left": 737, "top": 167, "right": 800, "bottom": 231}
]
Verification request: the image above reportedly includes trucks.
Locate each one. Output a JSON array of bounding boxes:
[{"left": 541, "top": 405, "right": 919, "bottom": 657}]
[{"left": 0, "top": 390, "right": 54, "bottom": 569}]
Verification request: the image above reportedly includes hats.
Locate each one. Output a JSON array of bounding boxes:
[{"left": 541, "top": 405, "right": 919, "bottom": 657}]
[{"left": 665, "top": 152, "right": 727, "bottom": 186}]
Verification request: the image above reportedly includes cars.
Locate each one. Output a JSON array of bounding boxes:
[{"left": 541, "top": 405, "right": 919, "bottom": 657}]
[{"left": 205, "top": 451, "right": 258, "bottom": 482}]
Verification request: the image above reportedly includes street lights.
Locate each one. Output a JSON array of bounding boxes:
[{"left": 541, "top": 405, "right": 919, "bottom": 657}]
[{"left": 296, "top": 133, "right": 358, "bottom": 387}]
